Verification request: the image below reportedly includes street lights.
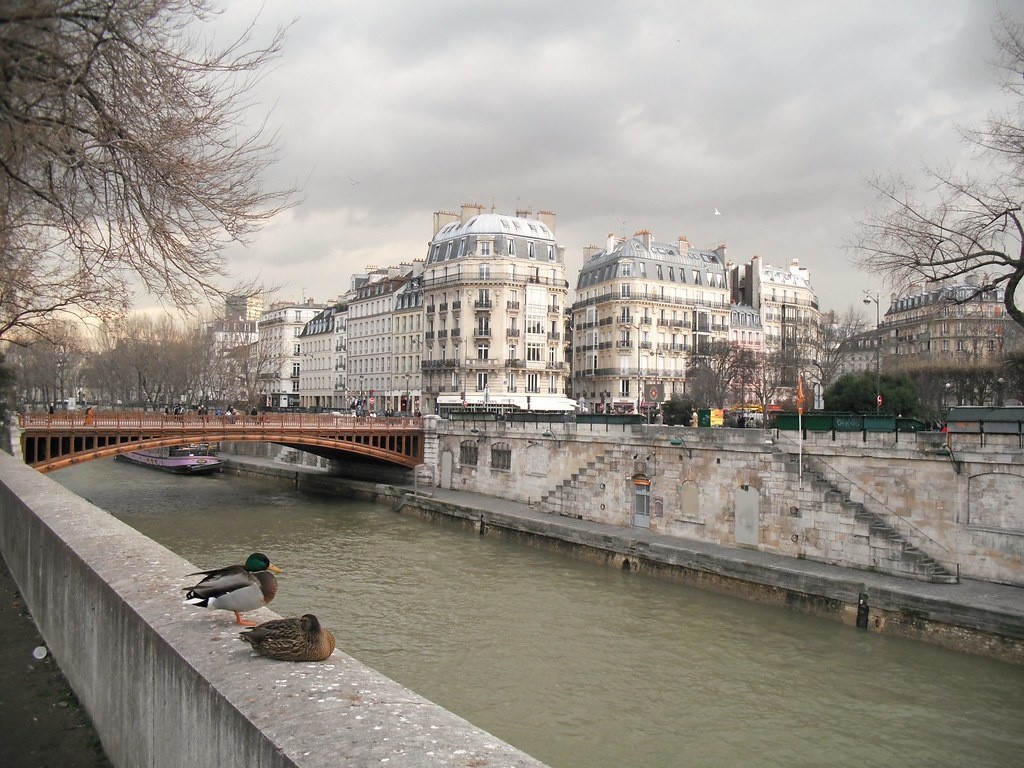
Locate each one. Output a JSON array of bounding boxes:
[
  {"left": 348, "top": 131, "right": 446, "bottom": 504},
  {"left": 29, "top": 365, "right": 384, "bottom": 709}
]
[
  {"left": 405, "top": 371, "right": 410, "bottom": 412},
  {"left": 624, "top": 322, "right": 641, "bottom": 414},
  {"left": 862, "top": 289, "right": 880, "bottom": 416}
]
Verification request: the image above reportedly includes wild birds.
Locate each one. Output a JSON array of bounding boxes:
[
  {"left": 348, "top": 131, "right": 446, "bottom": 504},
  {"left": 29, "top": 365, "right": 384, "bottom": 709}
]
[
  {"left": 237, "top": 612, "right": 336, "bottom": 663},
  {"left": 714, "top": 208, "right": 723, "bottom": 217},
  {"left": 182, "top": 552, "right": 286, "bottom": 627}
]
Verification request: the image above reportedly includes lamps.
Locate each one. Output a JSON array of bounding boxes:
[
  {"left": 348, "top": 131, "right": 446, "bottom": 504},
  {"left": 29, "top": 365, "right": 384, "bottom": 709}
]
[
  {"left": 936, "top": 444, "right": 961, "bottom": 476},
  {"left": 471, "top": 425, "right": 486, "bottom": 444},
  {"left": 543, "top": 430, "right": 561, "bottom": 449},
  {"left": 670, "top": 435, "right": 692, "bottom": 461}
]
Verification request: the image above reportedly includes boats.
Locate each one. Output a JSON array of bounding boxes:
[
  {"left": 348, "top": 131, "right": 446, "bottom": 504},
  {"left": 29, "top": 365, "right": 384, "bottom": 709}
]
[{"left": 112, "top": 440, "right": 225, "bottom": 477}]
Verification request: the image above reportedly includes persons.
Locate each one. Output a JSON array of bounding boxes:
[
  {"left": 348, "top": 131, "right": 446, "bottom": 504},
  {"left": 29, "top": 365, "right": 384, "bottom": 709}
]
[
  {"left": 245, "top": 407, "right": 265, "bottom": 425},
  {"left": 942, "top": 423, "right": 947, "bottom": 432},
  {"left": 165, "top": 406, "right": 168, "bottom": 422},
  {"left": 49, "top": 403, "right": 54, "bottom": 414},
  {"left": 197, "top": 405, "right": 209, "bottom": 423},
  {"left": 690, "top": 409, "right": 698, "bottom": 427},
  {"left": 385, "top": 409, "right": 394, "bottom": 426},
  {"left": 174, "top": 406, "right": 185, "bottom": 423},
  {"left": 418, "top": 411, "right": 421, "bottom": 417},
  {"left": 85, "top": 407, "right": 94, "bottom": 425},
  {"left": 738, "top": 415, "right": 745, "bottom": 427},
  {"left": 654, "top": 413, "right": 663, "bottom": 424},
  {"left": 216, "top": 405, "right": 240, "bottom": 424}
]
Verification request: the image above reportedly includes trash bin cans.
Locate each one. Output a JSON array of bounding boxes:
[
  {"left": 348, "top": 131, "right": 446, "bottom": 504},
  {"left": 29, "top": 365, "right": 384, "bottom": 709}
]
[
  {"left": 774, "top": 411, "right": 804, "bottom": 430},
  {"left": 833, "top": 412, "right": 864, "bottom": 432},
  {"left": 896, "top": 417, "right": 926, "bottom": 432},
  {"left": 698, "top": 409, "right": 711, "bottom": 427},
  {"left": 801, "top": 411, "right": 833, "bottom": 431},
  {"left": 864, "top": 413, "right": 897, "bottom": 432}
]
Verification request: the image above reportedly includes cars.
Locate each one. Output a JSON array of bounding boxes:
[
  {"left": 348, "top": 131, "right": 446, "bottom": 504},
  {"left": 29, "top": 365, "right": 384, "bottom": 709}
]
[
  {"left": 423, "top": 414, "right": 442, "bottom": 420},
  {"left": 392, "top": 411, "right": 413, "bottom": 418}
]
